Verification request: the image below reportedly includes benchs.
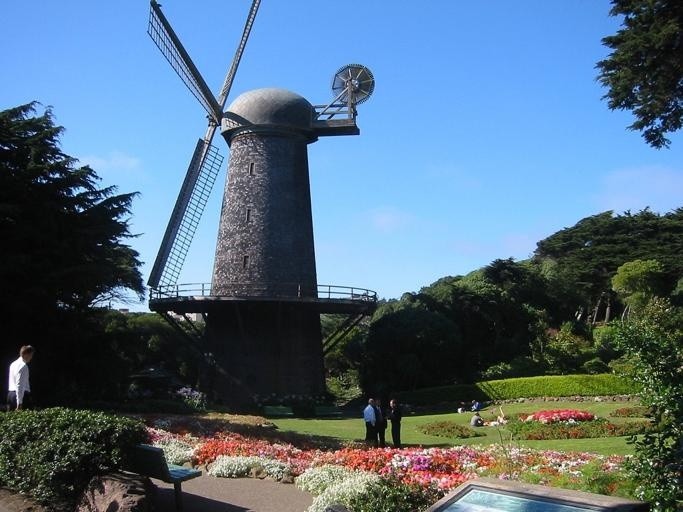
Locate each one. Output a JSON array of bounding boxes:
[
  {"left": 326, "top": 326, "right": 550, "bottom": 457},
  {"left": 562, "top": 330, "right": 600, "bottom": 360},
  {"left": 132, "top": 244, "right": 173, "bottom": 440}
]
[{"left": 121, "top": 444, "right": 202, "bottom": 511}]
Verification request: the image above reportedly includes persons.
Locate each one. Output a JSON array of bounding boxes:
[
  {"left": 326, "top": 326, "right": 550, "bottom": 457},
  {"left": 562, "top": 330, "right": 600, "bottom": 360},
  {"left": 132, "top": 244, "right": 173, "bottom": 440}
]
[
  {"left": 384, "top": 399, "right": 401, "bottom": 448},
  {"left": 373, "top": 398, "right": 387, "bottom": 448},
  {"left": 7, "top": 345, "right": 35, "bottom": 411},
  {"left": 457, "top": 400, "right": 510, "bottom": 428},
  {"left": 363, "top": 398, "right": 376, "bottom": 440}
]
